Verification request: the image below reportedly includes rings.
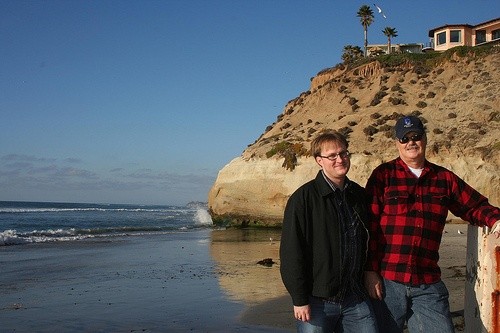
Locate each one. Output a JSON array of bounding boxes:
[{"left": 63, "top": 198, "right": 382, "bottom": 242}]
[{"left": 298, "top": 317, "right": 301, "bottom": 319}]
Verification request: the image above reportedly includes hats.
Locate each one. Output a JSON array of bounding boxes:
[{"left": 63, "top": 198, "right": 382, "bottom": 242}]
[{"left": 395, "top": 116, "right": 425, "bottom": 138}]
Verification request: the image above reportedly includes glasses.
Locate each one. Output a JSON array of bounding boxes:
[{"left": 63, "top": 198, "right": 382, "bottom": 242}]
[
  {"left": 396, "top": 132, "right": 425, "bottom": 143},
  {"left": 320, "top": 149, "right": 350, "bottom": 160}
]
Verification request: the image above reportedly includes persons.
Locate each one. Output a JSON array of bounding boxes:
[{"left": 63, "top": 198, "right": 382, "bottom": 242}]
[
  {"left": 365, "top": 116, "right": 500, "bottom": 333},
  {"left": 278, "top": 133, "right": 376, "bottom": 333}
]
